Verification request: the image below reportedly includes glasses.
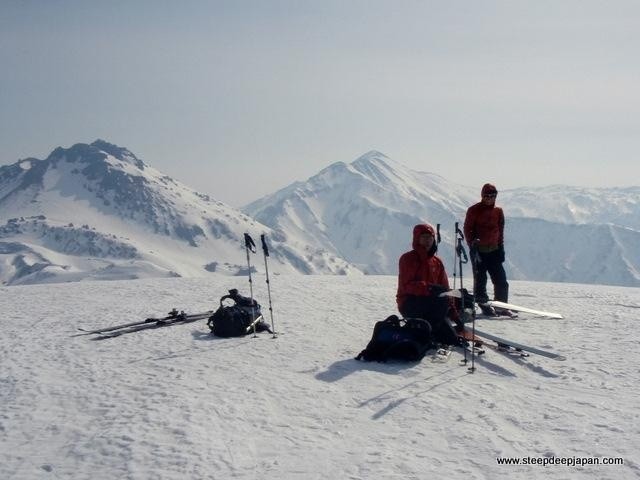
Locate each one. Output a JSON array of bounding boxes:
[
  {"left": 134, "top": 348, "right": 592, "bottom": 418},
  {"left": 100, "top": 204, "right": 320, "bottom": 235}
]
[{"left": 482, "top": 191, "right": 497, "bottom": 197}]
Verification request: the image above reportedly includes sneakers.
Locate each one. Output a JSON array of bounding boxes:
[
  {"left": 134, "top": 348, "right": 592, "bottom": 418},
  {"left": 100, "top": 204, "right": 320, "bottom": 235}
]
[
  {"left": 497, "top": 308, "right": 513, "bottom": 316},
  {"left": 483, "top": 307, "right": 496, "bottom": 316}
]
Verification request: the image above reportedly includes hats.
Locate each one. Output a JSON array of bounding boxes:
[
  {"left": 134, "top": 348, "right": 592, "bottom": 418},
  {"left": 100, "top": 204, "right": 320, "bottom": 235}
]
[{"left": 482, "top": 184, "right": 496, "bottom": 193}]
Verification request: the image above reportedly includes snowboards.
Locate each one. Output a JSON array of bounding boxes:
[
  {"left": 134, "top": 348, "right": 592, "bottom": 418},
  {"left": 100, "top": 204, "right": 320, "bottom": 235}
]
[{"left": 468, "top": 292, "right": 563, "bottom": 318}]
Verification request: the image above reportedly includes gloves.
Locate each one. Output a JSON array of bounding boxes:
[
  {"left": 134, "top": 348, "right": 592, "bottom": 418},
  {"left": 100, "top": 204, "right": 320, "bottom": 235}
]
[
  {"left": 471, "top": 244, "right": 478, "bottom": 254},
  {"left": 499, "top": 246, "right": 505, "bottom": 262},
  {"left": 428, "top": 285, "right": 448, "bottom": 296},
  {"left": 454, "top": 316, "right": 464, "bottom": 330}
]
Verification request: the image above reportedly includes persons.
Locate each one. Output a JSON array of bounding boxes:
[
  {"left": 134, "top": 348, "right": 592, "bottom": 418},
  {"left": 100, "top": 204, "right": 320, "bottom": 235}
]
[
  {"left": 461, "top": 182, "right": 518, "bottom": 319},
  {"left": 395, "top": 223, "right": 469, "bottom": 348}
]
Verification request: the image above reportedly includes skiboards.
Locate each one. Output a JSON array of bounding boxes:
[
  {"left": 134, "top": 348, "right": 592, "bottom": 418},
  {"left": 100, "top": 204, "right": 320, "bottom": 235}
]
[
  {"left": 77, "top": 310, "right": 216, "bottom": 338},
  {"left": 450, "top": 320, "right": 567, "bottom": 362}
]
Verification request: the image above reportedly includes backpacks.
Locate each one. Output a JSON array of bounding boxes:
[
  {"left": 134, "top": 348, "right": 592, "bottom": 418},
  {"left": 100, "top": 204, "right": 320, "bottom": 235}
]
[
  {"left": 207, "top": 288, "right": 273, "bottom": 337},
  {"left": 355, "top": 315, "right": 435, "bottom": 361}
]
